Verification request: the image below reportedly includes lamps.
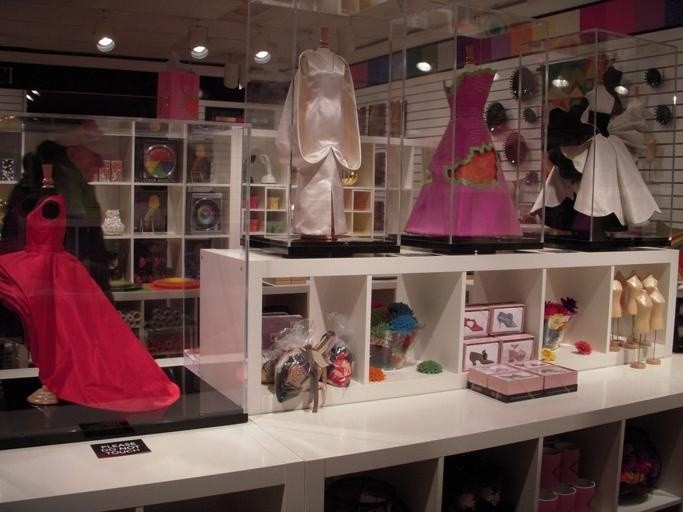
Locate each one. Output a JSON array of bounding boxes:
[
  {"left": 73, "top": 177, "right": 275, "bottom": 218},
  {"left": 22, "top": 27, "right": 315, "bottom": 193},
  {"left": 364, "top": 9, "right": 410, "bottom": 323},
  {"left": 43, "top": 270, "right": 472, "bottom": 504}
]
[
  {"left": 614, "top": 79, "right": 630, "bottom": 95},
  {"left": 551, "top": 73, "right": 569, "bottom": 88},
  {"left": 94, "top": 7, "right": 271, "bottom": 64}
]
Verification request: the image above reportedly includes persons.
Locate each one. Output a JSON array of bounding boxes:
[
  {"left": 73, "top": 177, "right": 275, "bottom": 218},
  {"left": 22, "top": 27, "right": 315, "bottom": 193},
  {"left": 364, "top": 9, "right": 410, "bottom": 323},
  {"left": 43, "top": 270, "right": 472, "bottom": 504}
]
[
  {"left": 53, "top": 129, "right": 116, "bottom": 309},
  {"left": 259, "top": 154, "right": 278, "bottom": 185},
  {"left": 271, "top": 28, "right": 362, "bottom": 240},
  {"left": 0, "top": 160, "right": 183, "bottom": 413},
  {"left": 612, "top": 269, "right": 667, "bottom": 369},
  {"left": 404, "top": 55, "right": 523, "bottom": 238},
  {"left": 530, "top": 65, "right": 662, "bottom": 241},
  {"left": 583, "top": 64, "right": 630, "bottom": 152}
]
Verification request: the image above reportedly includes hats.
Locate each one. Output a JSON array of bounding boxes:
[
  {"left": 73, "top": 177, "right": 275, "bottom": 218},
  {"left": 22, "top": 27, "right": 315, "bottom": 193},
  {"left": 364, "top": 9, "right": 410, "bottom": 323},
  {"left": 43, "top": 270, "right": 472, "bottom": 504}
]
[{"left": 56, "top": 120, "right": 115, "bottom": 156}]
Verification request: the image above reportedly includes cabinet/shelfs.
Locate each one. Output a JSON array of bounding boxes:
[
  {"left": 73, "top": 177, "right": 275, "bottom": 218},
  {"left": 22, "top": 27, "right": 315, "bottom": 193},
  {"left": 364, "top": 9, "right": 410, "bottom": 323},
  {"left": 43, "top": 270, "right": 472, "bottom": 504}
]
[
  {"left": 238, "top": 123, "right": 439, "bottom": 241},
  {"left": 199, "top": 244, "right": 679, "bottom": 428},
  {"left": 0, "top": 98, "right": 239, "bottom": 355},
  {"left": 4, "top": 350, "right": 683, "bottom": 512}
]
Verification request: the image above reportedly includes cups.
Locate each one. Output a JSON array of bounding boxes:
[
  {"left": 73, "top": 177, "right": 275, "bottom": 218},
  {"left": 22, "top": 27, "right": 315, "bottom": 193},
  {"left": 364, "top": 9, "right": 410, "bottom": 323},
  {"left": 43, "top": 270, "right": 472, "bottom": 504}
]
[{"left": 539, "top": 440, "right": 596, "bottom": 511}]
